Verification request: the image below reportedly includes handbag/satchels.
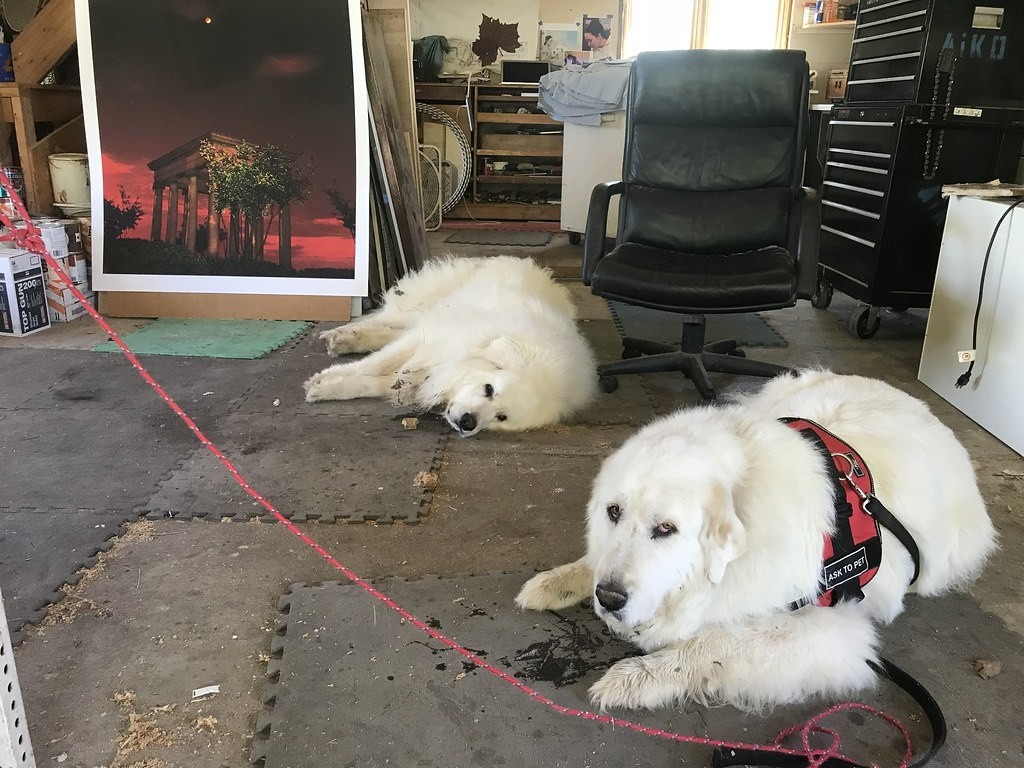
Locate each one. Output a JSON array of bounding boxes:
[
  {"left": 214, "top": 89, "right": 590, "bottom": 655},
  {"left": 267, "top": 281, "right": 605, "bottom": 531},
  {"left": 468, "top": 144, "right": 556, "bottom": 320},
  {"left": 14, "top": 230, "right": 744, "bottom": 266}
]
[{"left": 413, "top": 35, "right": 450, "bottom": 82}]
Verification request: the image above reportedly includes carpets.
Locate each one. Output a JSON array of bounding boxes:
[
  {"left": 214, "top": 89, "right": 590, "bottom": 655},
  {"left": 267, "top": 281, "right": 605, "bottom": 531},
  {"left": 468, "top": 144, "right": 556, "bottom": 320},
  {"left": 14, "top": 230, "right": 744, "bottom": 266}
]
[{"left": 444, "top": 229, "right": 553, "bottom": 246}]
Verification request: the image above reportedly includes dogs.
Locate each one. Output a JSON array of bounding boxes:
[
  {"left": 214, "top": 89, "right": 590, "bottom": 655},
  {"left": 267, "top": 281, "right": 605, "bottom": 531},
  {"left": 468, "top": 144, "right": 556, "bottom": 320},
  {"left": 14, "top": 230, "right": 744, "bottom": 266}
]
[
  {"left": 512, "top": 365, "right": 1008, "bottom": 717},
  {"left": 301, "top": 253, "right": 604, "bottom": 438}
]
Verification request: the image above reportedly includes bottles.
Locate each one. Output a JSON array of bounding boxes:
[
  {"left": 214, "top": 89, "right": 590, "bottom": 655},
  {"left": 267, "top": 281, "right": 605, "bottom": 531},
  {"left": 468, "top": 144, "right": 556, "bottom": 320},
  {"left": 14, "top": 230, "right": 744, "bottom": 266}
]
[{"left": 815, "top": 0, "right": 839, "bottom": 24}]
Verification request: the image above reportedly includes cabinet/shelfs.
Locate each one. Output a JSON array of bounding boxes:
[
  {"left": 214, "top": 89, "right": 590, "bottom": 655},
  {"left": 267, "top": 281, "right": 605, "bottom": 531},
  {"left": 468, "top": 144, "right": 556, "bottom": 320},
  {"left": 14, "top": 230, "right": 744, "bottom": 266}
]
[
  {"left": 811, "top": 0, "right": 1024, "bottom": 338},
  {"left": 412, "top": 82, "right": 565, "bottom": 220}
]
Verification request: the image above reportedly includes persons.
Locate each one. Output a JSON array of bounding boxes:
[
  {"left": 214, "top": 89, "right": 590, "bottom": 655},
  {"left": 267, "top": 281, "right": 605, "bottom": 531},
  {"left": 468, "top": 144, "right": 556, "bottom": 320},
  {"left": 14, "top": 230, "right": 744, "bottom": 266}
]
[
  {"left": 541, "top": 36, "right": 555, "bottom": 62},
  {"left": 584, "top": 21, "right": 612, "bottom": 63}
]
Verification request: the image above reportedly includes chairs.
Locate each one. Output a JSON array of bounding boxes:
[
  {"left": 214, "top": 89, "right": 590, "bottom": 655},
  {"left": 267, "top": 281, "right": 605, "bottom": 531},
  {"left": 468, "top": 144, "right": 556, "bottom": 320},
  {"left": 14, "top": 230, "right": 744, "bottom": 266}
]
[{"left": 581, "top": 50, "right": 837, "bottom": 402}]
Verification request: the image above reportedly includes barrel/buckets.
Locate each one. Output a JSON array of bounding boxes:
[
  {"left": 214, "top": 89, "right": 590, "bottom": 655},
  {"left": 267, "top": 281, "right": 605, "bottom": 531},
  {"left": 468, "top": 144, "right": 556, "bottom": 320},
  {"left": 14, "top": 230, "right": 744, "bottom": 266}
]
[
  {"left": 46, "top": 153, "right": 90, "bottom": 205},
  {"left": 1, "top": 166, "right": 23, "bottom": 197},
  {"left": 13, "top": 216, "right": 89, "bottom": 284}
]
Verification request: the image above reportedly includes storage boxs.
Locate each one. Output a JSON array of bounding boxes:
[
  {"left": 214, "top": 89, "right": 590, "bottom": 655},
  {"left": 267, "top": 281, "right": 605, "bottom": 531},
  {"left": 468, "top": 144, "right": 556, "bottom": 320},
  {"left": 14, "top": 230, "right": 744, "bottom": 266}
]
[{"left": 0, "top": 217, "right": 94, "bottom": 337}]
